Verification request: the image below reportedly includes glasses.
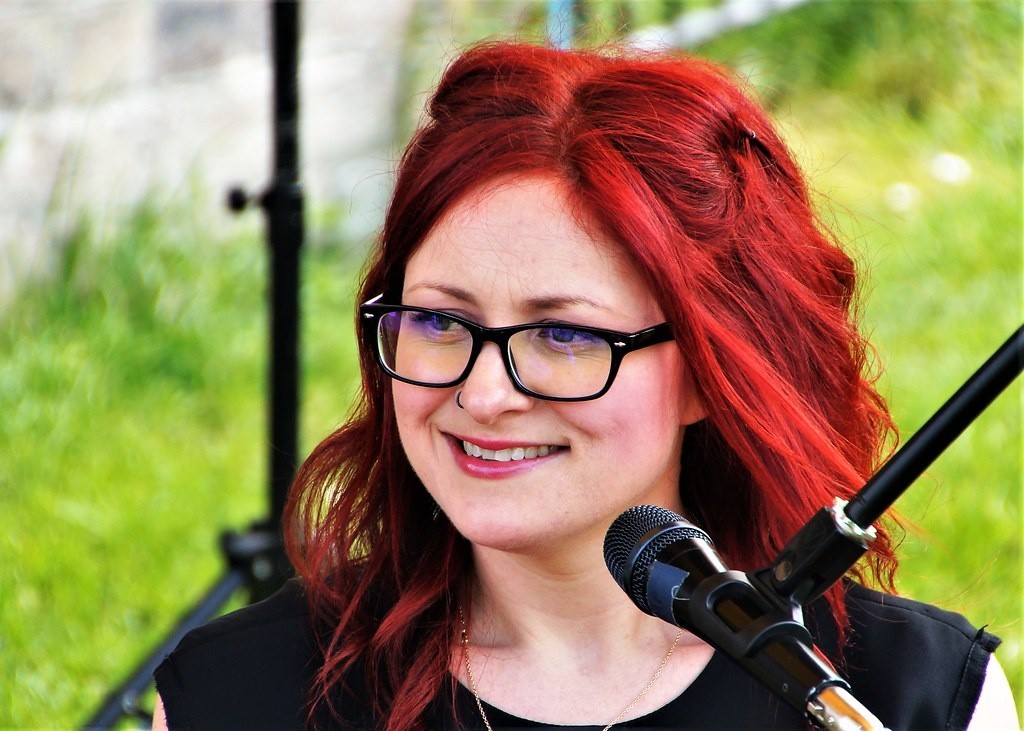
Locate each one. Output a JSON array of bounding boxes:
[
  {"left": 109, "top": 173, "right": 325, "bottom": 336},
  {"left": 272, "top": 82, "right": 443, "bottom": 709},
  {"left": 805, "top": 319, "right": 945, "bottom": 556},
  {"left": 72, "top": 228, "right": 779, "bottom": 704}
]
[{"left": 359, "top": 291, "right": 676, "bottom": 402}]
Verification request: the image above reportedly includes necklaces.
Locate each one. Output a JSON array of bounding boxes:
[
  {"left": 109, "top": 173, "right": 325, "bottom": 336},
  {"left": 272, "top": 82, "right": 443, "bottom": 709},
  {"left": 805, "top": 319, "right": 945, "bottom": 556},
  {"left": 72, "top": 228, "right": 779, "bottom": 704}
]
[{"left": 459, "top": 607, "right": 682, "bottom": 731}]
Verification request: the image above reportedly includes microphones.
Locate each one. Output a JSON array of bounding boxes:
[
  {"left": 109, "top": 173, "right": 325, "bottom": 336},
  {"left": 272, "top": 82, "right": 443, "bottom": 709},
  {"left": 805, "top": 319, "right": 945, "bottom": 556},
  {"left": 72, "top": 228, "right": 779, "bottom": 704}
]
[{"left": 604, "top": 505, "right": 890, "bottom": 731}]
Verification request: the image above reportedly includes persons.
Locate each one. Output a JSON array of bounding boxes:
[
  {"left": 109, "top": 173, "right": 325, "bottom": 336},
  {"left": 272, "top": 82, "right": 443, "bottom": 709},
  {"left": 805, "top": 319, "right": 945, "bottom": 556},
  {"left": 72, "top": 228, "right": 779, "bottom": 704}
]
[{"left": 155, "top": 43, "right": 1021, "bottom": 731}]
